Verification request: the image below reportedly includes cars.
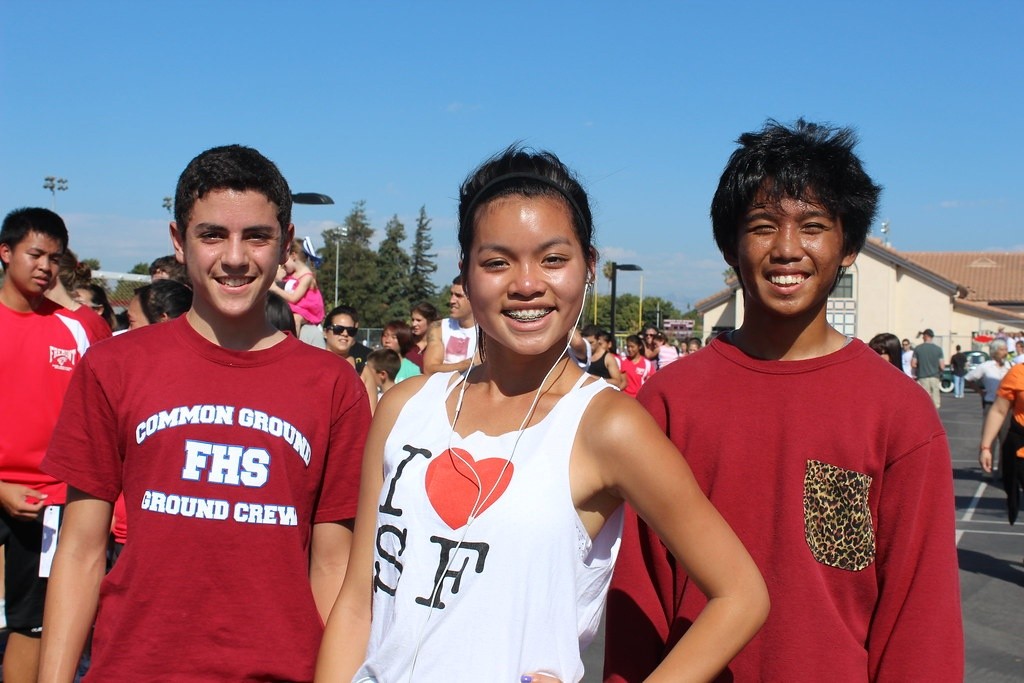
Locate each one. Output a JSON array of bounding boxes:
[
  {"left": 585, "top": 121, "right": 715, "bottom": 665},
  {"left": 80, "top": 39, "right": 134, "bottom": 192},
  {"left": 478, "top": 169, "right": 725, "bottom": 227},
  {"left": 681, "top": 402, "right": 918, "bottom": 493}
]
[{"left": 939, "top": 350, "right": 993, "bottom": 395}]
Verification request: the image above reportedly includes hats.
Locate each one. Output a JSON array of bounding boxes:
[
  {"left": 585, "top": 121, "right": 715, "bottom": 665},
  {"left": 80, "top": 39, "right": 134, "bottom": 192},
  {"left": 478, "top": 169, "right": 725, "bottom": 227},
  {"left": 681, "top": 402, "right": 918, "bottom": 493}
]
[{"left": 920, "top": 329, "right": 934, "bottom": 337}]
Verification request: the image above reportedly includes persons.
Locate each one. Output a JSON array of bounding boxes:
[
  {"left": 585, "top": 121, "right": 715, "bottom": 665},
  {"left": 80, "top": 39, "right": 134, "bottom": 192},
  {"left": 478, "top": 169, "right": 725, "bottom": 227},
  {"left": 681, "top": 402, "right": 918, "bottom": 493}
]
[
  {"left": 35, "top": 144, "right": 372, "bottom": 683},
  {"left": 901, "top": 339, "right": 917, "bottom": 379},
  {"left": 950, "top": 345, "right": 969, "bottom": 398},
  {"left": 979, "top": 363, "right": 1023, "bottom": 527},
  {"left": 963, "top": 327, "right": 1024, "bottom": 483},
  {"left": 315, "top": 152, "right": 771, "bottom": 683},
  {"left": 869, "top": 333, "right": 904, "bottom": 372},
  {"left": 45, "top": 239, "right": 717, "bottom": 571},
  {"left": 0, "top": 206, "right": 98, "bottom": 683},
  {"left": 911, "top": 329, "right": 945, "bottom": 408},
  {"left": 602, "top": 119, "right": 965, "bottom": 683}
]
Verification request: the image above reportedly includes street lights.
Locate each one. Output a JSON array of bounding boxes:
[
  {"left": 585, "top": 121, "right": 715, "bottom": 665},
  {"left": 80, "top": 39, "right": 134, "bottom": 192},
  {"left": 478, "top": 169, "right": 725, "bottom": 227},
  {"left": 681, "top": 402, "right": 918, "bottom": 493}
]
[
  {"left": 327, "top": 240, "right": 340, "bottom": 308},
  {"left": 609, "top": 261, "right": 643, "bottom": 354},
  {"left": 163, "top": 196, "right": 178, "bottom": 257},
  {"left": 42, "top": 176, "right": 69, "bottom": 213}
]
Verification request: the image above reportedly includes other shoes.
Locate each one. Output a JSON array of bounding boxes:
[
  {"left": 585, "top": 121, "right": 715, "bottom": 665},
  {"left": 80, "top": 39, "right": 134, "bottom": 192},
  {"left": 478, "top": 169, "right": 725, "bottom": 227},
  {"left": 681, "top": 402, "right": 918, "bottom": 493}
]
[
  {"left": 959, "top": 395, "right": 963, "bottom": 398},
  {"left": 954, "top": 395, "right": 958, "bottom": 398}
]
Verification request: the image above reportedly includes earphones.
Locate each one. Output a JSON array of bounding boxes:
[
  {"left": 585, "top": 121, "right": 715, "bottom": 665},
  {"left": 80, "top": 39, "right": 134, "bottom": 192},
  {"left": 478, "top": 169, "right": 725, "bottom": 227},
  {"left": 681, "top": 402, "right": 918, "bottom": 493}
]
[{"left": 584, "top": 266, "right": 591, "bottom": 287}]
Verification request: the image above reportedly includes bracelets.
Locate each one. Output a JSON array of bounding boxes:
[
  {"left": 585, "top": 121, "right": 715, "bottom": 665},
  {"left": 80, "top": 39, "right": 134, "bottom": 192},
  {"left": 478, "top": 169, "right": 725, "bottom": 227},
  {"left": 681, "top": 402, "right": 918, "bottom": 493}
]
[
  {"left": 470, "top": 358, "right": 474, "bottom": 366},
  {"left": 980, "top": 445, "right": 991, "bottom": 450}
]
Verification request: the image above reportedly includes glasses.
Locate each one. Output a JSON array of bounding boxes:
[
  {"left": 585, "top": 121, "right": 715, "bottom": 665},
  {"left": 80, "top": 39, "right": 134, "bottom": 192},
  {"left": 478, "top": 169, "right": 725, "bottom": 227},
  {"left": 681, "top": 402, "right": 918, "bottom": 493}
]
[
  {"left": 646, "top": 334, "right": 655, "bottom": 337},
  {"left": 326, "top": 324, "right": 358, "bottom": 337}
]
